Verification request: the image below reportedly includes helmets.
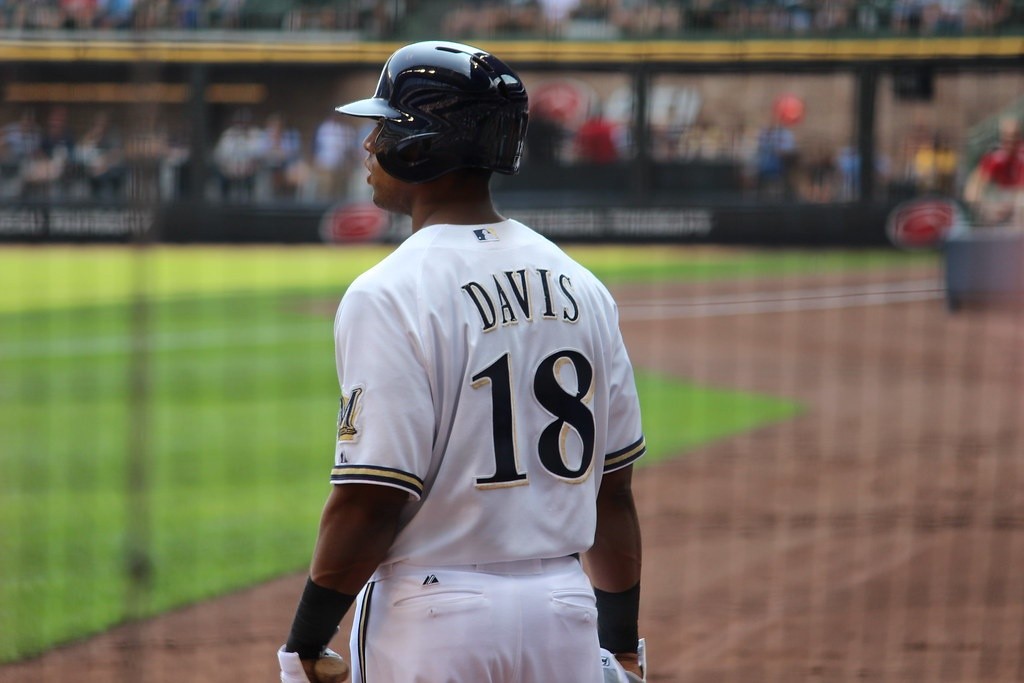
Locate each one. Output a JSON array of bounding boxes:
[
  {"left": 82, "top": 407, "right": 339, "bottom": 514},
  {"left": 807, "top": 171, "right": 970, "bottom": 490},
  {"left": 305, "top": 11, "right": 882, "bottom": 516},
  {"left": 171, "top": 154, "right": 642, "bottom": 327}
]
[{"left": 334, "top": 40, "right": 531, "bottom": 184}]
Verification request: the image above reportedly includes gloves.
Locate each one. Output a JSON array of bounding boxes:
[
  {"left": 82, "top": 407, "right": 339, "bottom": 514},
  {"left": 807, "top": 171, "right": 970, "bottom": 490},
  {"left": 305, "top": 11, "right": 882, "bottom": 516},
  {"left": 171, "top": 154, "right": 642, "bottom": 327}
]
[
  {"left": 275, "top": 643, "right": 348, "bottom": 683},
  {"left": 599, "top": 637, "right": 647, "bottom": 683}
]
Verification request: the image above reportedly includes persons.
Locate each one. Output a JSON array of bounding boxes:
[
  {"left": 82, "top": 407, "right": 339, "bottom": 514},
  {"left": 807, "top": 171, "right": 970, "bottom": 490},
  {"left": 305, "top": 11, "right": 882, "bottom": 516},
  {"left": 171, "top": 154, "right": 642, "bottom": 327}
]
[
  {"left": 0, "top": 0, "right": 1024, "bottom": 230},
  {"left": 276, "top": 41, "right": 647, "bottom": 683}
]
